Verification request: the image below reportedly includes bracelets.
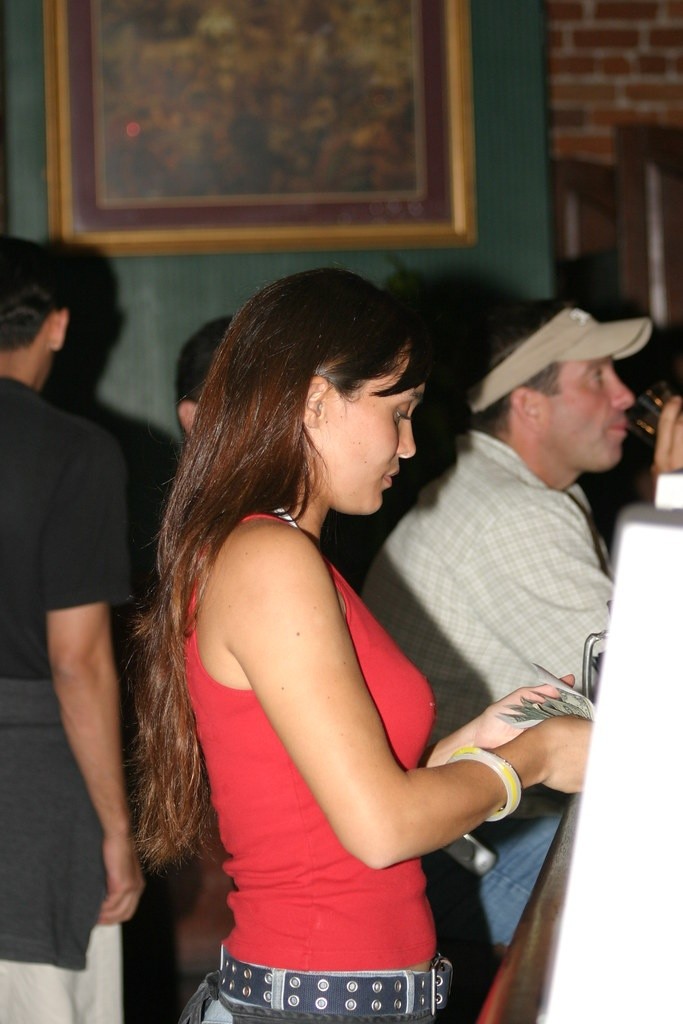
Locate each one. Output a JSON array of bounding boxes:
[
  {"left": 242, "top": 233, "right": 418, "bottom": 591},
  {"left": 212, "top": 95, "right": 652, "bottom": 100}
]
[{"left": 446, "top": 747, "right": 522, "bottom": 822}]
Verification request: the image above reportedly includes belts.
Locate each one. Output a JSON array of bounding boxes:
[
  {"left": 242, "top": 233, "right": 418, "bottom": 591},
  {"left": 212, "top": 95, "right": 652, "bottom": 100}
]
[{"left": 216, "top": 945, "right": 454, "bottom": 1016}]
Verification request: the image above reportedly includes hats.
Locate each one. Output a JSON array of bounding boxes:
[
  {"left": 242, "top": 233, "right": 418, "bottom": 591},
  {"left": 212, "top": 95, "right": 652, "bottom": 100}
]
[{"left": 467, "top": 307, "right": 653, "bottom": 413}]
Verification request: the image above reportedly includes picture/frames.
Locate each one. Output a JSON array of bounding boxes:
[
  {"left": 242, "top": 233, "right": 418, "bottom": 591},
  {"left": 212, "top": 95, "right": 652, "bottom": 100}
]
[{"left": 43, "top": 0, "right": 480, "bottom": 256}]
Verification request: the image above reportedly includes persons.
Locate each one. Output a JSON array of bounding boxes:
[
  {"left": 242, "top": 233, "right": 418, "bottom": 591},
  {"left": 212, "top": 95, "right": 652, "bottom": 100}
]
[
  {"left": 131, "top": 267, "right": 595, "bottom": 1024},
  {"left": 362, "top": 297, "right": 682, "bottom": 948},
  {"left": 0, "top": 234, "right": 161, "bottom": 1024}
]
